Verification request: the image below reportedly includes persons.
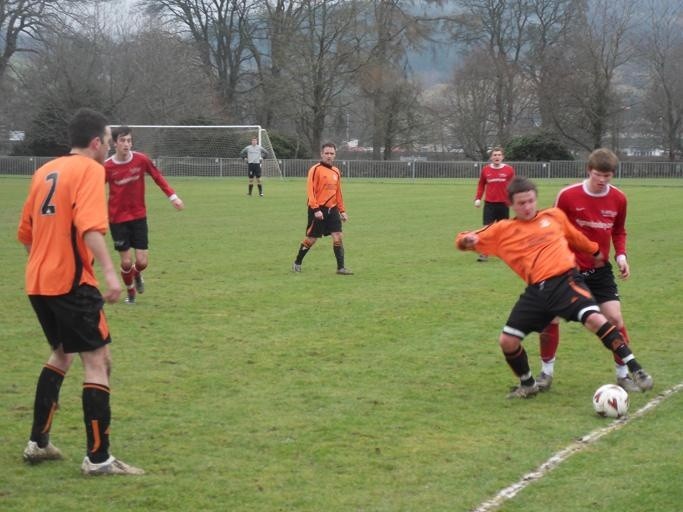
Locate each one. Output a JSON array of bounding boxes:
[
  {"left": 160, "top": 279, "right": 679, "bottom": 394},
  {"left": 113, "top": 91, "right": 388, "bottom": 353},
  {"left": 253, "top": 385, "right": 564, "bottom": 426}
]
[
  {"left": 93, "top": 127, "right": 183, "bottom": 305},
  {"left": 239, "top": 138, "right": 269, "bottom": 195},
  {"left": 470, "top": 147, "right": 517, "bottom": 263},
  {"left": 536, "top": 147, "right": 645, "bottom": 393},
  {"left": 290, "top": 143, "right": 356, "bottom": 276},
  {"left": 16, "top": 107, "right": 146, "bottom": 479},
  {"left": 454, "top": 175, "right": 653, "bottom": 398}
]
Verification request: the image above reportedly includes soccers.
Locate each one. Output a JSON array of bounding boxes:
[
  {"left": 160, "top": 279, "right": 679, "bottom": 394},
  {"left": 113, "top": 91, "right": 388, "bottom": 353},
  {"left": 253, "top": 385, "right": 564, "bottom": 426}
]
[{"left": 593, "top": 383, "right": 629, "bottom": 418}]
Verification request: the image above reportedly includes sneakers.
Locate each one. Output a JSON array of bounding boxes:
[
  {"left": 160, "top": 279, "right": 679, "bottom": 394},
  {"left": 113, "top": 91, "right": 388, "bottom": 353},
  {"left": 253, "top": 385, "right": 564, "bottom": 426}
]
[
  {"left": 507, "top": 369, "right": 656, "bottom": 398},
  {"left": 128, "top": 287, "right": 136, "bottom": 303},
  {"left": 336, "top": 268, "right": 354, "bottom": 275},
  {"left": 134, "top": 275, "right": 144, "bottom": 294},
  {"left": 477, "top": 256, "right": 488, "bottom": 261},
  {"left": 80, "top": 456, "right": 145, "bottom": 476},
  {"left": 23, "top": 440, "right": 63, "bottom": 462},
  {"left": 295, "top": 264, "right": 301, "bottom": 272}
]
